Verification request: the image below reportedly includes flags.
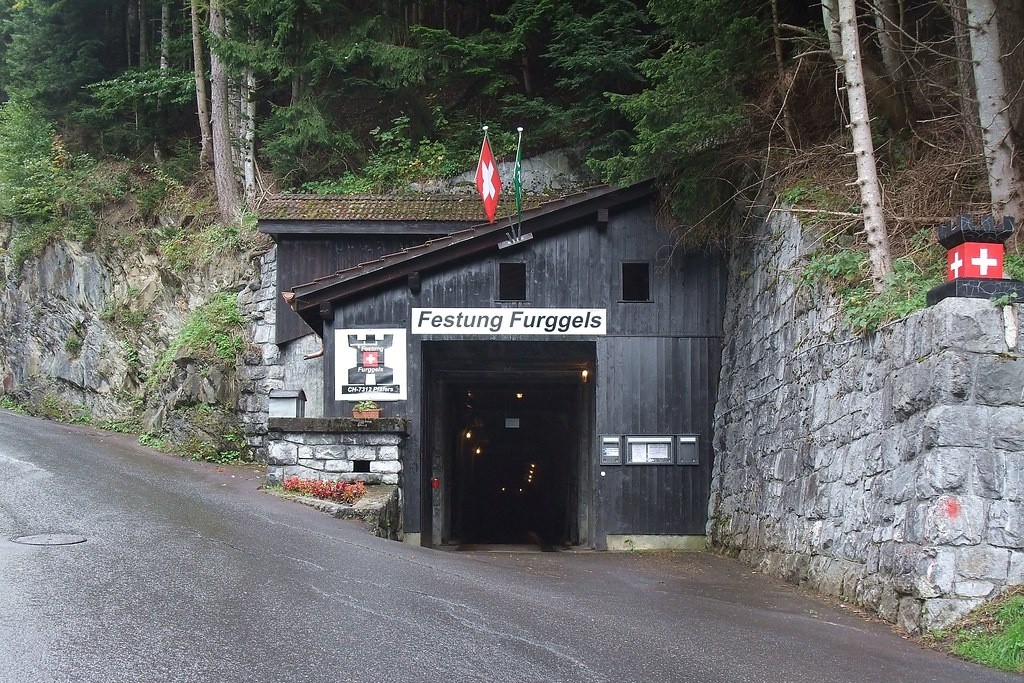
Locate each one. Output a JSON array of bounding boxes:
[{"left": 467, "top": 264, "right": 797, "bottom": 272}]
[
  {"left": 512, "top": 132, "right": 523, "bottom": 226},
  {"left": 472, "top": 133, "right": 503, "bottom": 226}
]
